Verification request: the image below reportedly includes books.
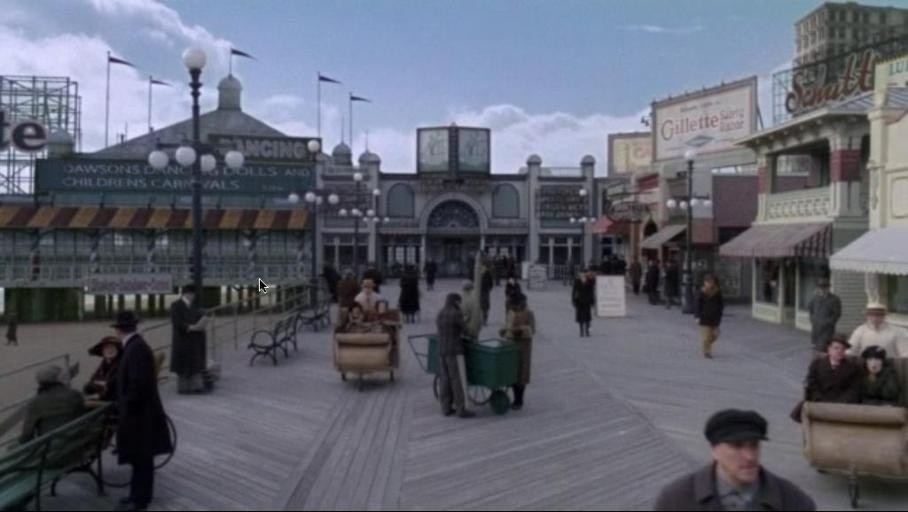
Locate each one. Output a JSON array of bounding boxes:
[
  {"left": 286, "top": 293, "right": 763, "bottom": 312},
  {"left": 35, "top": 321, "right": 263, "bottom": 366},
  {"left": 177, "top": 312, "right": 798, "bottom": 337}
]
[{"left": 195, "top": 311, "right": 217, "bottom": 331}]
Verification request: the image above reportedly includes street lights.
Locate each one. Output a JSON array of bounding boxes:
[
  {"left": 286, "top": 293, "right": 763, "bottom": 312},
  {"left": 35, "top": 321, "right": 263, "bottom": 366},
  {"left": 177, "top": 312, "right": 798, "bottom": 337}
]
[
  {"left": 146, "top": 45, "right": 243, "bottom": 390},
  {"left": 569, "top": 187, "right": 597, "bottom": 273},
  {"left": 364, "top": 189, "right": 390, "bottom": 270},
  {"left": 340, "top": 168, "right": 364, "bottom": 292},
  {"left": 288, "top": 138, "right": 339, "bottom": 314},
  {"left": 666, "top": 148, "right": 712, "bottom": 314}
]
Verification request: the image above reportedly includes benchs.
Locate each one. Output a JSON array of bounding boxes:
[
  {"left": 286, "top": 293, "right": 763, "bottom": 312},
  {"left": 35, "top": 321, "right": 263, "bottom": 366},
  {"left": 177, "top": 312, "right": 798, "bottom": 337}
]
[
  {"left": 1, "top": 403, "right": 117, "bottom": 511},
  {"left": 299, "top": 296, "right": 334, "bottom": 333},
  {"left": 245, "top": 312, "right": 302, "bottom": 366}
]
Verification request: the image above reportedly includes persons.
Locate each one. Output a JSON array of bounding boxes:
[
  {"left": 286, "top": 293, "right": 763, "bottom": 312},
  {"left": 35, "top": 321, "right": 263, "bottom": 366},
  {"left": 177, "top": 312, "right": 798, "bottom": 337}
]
[
  {"left": 465, "top": 249, "right": 520, "bottom": 324},
  {"left": 857, "top": 343, "right": 907, "bottom": 403},
  {"left": 2, "top": 300, "right": 22, "bottom": 345},
  {"left": 423, "top": 257, "right": 438, "bottom": 292},
  {"left": 319, "top": 259, "right": 420, "bottom": 333},
  {"left": 790, "top": 331, "right": 864, "bottom": 426},
  {"left": 101, "top": 309, "right": 174, "bottom": 512},
  {"left": 498, "top": 294, "right": 535, "bottom": 411},
  {"left": 805, "top": 275, "right": 844, "bottom": 353},
  {"left": 434, "top": 292, "right": 475, "bottom": 418},
  {"left": 845, "top": 298, "right": 907, "bottom": 361},
  {"left": 17, "top": 364, "right": 87, "bottom": 468},
  {"left": 652, "top": 406, "right": 819, "bottom": 510},
  {"left": 170, "top": 283, "right": 204, "bottom": 395},
  {"left": 600, "top": 252, "right": 680, "bottom": 308},
  {"left": 694, "top": 272, "right": 723, "bottom": 356},
  {"left": 572, "top": 269, "right": 596, "bottom": 335},
  {"left": 85, "top": 335, "right": 127, "bottom": 403}
]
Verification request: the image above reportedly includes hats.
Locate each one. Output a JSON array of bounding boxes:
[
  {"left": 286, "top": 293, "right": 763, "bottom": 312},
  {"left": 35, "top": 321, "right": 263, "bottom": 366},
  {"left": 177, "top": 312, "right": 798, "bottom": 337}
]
[
  {"left": 110, "top": 309, "right": 139, "bottom": 328},
  {"left": 37, "top": 364, "right": 60, "bottom": 383},
  {"left": 862, "top": 302, "right": 890, "bottom": 316},
  {"left": 825, "top": 331, "right": 851, "bottom": 348},
  {"left": 705, "top": 409, "right": 769, "bottom": 443},
  {"left": 360, "top": 280, "right": 375, "bottom": 288},
  {"left": 89, "top": 337, "right": 121, "bottom": 354},
  {"left": 862, "top": 346, "right": 887, "bottom": 360}
]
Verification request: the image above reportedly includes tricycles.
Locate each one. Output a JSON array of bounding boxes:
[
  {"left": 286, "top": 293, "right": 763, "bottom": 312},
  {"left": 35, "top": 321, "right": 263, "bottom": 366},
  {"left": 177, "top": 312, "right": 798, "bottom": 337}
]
[
  {"left": 802, "top": 355, "right": 908, "bottom": 510},
  {"left": 408, "top": 334, "right": 521, "bottom": 415},
  {"left": 333, "top": 297, "right": 404, "bottom": 392}
]
